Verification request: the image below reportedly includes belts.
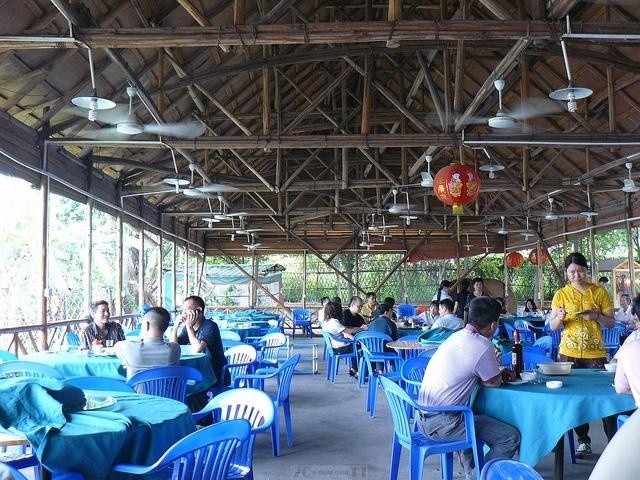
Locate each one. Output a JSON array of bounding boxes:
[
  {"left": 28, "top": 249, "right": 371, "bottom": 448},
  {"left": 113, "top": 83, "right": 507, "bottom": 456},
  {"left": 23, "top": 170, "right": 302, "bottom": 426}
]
[{"left": 417, "top": 412, "right": 441, "bottom": 418}]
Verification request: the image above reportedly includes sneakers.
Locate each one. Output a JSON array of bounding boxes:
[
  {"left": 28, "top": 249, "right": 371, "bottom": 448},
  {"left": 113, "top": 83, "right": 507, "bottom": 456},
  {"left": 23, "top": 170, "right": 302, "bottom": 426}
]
[{"left": 575, "top": 443, "right": 592, "bottom": 458}]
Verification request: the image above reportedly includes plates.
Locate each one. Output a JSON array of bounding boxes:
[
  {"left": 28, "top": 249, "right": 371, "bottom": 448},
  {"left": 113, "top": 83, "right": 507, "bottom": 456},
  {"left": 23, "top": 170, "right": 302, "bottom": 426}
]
[
  {"left": 84, "top": 393, "right": 116, "bottom": 410},
  {"left": 507, "top": 380, "right": 528, "bottom": 385}
]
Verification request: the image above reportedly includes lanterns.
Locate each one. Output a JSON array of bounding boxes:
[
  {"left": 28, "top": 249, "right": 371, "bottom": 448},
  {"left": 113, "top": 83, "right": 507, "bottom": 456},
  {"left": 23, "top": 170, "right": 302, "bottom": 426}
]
[
  {"left": 530, "top": 248, "right": 549, "bottom": 266},
  {"left": 433, "top": 163, "right": 480, "bottom": 241},
  {"left": 506, "top": 252, "right": 524, "bottom": 270}
]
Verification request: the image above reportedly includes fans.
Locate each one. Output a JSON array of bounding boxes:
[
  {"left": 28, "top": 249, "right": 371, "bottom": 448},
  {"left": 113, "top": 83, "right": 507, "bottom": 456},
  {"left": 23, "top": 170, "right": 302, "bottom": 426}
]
[
  {"left": 481, "top": 215, "right": 529, "bottom": 238},
  {"left": 390, "top": 154, "right": 437, "bottom": 190},
  {"left": 422, "top": 78, "right": 558, "bottom": 139},
  {"left": 534, "top": 198, "right": 580, "bottom": 223},
  {"left": 587, "top": 157, "right": 640, "bottom": 198},
  {"left": 59, "top": 87, "right": 268, "bottom": 258},
  {"left": 359, "top": 188, "right": 423, "bottom": 219}
]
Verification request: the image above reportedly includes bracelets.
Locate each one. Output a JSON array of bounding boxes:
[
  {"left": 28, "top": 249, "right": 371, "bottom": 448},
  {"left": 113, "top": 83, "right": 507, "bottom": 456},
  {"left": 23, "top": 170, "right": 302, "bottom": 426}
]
[{"left": 464, "top": 321, "right": 467, "bottom": 324}]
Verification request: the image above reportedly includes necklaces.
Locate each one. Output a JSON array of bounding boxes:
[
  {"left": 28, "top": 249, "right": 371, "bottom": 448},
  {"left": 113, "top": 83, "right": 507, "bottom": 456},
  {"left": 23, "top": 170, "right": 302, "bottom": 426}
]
[{"left": 598, "top": 283, "right": 601, "bottom": 287}]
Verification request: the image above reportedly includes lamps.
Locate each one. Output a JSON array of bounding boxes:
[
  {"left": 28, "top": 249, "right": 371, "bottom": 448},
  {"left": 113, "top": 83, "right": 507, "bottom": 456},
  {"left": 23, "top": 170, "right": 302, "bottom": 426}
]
[
  {"left": 397, "top": 210, "right": 420, "bottom": 227},
  {"left": 475, "top": 157, "right": 507, "bottom": 182},
  {"left": 519, "top": 228, "right": 537, "bottom": 241},
  {"left": 578, "top": 198, "right": 601, "bottom": 224},
  {"left": 70, "top": 49, "right": 117, "bottom": 122},
  {"left": 548, "top": 34, "right": 594, "bottom": 112}
]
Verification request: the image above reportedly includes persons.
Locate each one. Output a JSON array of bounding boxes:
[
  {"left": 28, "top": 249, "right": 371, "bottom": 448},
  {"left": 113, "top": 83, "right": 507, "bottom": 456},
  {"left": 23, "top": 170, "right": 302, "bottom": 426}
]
[
  {"left": 549, "top": 252, "right": 616, "bottom": 458},
  {"left": 614, "top": 293, "right": 639, "bottom": 408},
  {"left": 318, "top": 292, "right": 396, "bottom": 337},
  {"left": 418, "top": 296, "right": 520, "bottom": 480},
  {"left": 407, "top": 299, "right": 440, "bottom": 329},
  {"left": 323, "top": 301, "right": 358, "bottom": 378},
  {"left": 79, "top": 299, "right": 126, "bottom": 349},
  {"left": 437, "top": 280, "right": 461, "bottom": 304},
  {"left": 453, "top": 280, "right": 470, "bottom": 319},
  {"left": 615, "top": 294, "right": 639, "bottom": 346},
  {"left": 115, "top": 307, "right": 181, "bottom": 394},
  {"left": 496, "top": 297, "right": 512, "bottom": 315},
  {"left": 463, "top": 278, "right": 491, "bottom": 328},
  {"left": 366, "top": 303, "right": 397, "bottom": 375},
  {"left": 523, "top": 299, "right": 545, "bottom": 339},
  {"left": 597, "top": 277, "right": 609, "bottom": 288},
  {"left": 170, "top": 296, "right": 230, "bottom": 427},
  {"left": 431, "top": 299, "right": 464, "bottom": 329}
]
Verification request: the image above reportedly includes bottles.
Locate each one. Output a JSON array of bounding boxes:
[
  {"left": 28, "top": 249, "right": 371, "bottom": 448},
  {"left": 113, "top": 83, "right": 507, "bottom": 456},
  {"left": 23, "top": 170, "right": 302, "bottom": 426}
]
[{"left": 511, "top": 329, "right": 524, "bottom": 380}]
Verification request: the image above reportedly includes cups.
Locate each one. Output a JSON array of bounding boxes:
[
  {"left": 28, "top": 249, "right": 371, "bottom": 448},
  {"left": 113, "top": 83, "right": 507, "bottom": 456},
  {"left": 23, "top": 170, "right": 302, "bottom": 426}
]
[
  {"left": 106, "top": 339, "right": 114, "bottom": 348},
  {"left": 93, "top": 345, "right": 103, "bottom": 356}
]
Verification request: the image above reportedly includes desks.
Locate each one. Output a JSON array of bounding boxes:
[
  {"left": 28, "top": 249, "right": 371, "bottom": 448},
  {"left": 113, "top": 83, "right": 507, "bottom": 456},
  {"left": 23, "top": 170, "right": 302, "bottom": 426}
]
[
  {"left": 0, "top": 391, "right": 191, "bottom": 480},
  {"left": 474, "top": 364, "right": 637, "bottom": 479}
]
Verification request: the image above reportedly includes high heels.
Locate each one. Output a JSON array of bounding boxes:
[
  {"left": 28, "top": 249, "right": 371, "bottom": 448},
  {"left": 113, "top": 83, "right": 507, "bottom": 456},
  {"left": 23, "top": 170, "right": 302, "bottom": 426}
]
[{"left": 348, "top": 370, "right": 356, "bottom": 378}]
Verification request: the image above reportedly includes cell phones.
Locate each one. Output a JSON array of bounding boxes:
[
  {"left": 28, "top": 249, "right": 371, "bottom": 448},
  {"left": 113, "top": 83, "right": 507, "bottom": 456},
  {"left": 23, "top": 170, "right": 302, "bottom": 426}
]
[{"left": 194, "top": 309, "right": 200, "bottom": 314}]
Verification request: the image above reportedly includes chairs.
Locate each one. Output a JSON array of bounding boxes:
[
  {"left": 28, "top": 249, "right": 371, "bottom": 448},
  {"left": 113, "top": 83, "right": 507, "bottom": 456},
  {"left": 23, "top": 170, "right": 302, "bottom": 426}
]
[
  {"left": 126, "top": 365, "right": 206, "bottom": 403},
  {"left": 291, "top": 306, "right": 314, "bottom": 343},
  {"left": 321, "top": 301, "right": 632, "bottom": 387},
  {"left": 375, "top": 374, "right": 483, "bottom": 480},
  {"left": 232, "top": 353, "right": 300, "bottom": 457},
  {"left": 65, "top": 305, "right": 286, "bottom": 389},
  {"left": 0, "top": 347, "right": 137, "bottom": 395},
  {"left": 355, "top": 337, "right": 431, "bottom": 423},
  {"left": 191, "top": 388, "right": 276, "bottom": 445},
  {"left": 402, "top": 345, "right": 632, "bottom": 466},
  {"left": 111, "top": 417, "right": 253, "bottom": 480}
]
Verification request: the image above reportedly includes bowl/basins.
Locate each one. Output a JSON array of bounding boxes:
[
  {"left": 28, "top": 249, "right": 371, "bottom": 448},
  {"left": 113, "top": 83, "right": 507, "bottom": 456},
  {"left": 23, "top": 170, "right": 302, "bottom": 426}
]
[
  {"left": 520, "top": 372, "right": 537, "bottom": 381},
  {"left": 604, "top": 363, "right": 617, "bottom": 372},
  {"left": 535, "top": 362, "right": 576, "bottom": 374}
]
